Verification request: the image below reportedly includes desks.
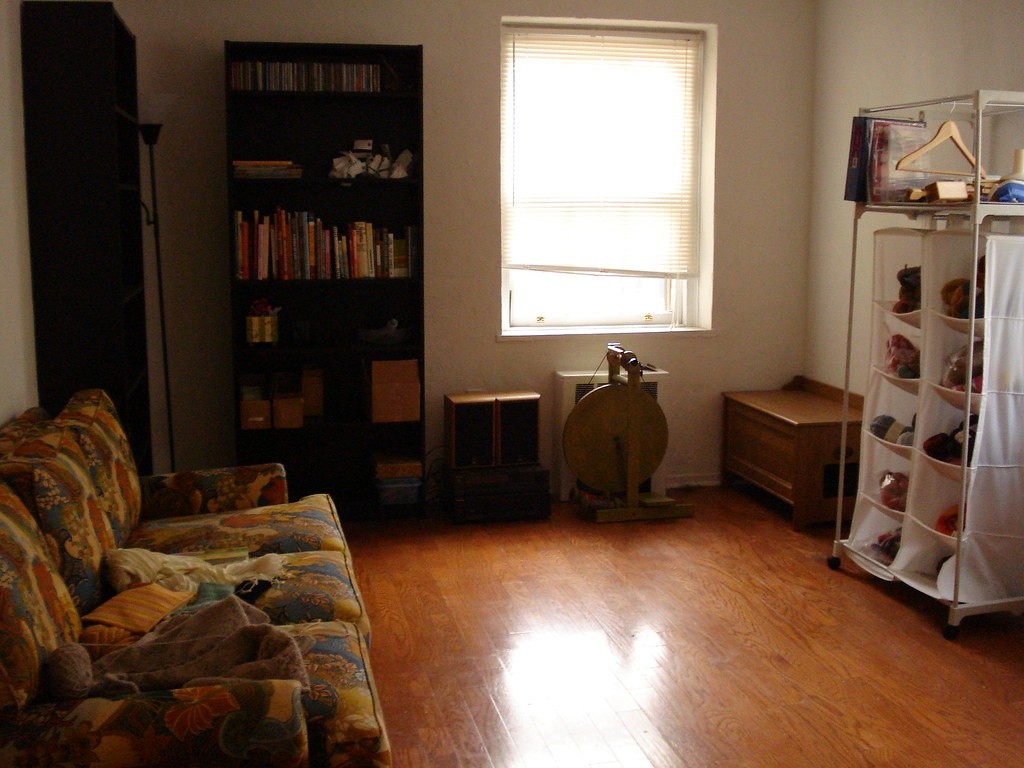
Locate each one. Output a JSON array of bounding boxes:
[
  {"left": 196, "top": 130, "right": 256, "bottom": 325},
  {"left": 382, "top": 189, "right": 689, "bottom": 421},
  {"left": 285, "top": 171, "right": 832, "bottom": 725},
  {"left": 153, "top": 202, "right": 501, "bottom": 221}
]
[{"left": 723, "top": 375, "right": 862, "bottom": 532}]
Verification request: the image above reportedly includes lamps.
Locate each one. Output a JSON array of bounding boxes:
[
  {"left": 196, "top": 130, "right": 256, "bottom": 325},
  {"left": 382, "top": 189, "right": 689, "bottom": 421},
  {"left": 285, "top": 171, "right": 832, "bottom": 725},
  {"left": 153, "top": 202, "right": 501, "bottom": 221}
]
[{"left": 140, "top": 124, "right": 175, "bottom": 472}]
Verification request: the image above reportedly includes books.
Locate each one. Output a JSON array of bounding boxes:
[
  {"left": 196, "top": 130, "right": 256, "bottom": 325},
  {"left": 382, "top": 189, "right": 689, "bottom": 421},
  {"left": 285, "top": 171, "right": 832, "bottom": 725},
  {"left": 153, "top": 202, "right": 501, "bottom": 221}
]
[
  {"left": 234, "top": 207, "right": 412, "bottom": 281},
  {"left": 234, "top": 159, "right": 303, "bottom": 180},
  {"left": 231, "top": 61, "right": 381, "bottom": 92}
]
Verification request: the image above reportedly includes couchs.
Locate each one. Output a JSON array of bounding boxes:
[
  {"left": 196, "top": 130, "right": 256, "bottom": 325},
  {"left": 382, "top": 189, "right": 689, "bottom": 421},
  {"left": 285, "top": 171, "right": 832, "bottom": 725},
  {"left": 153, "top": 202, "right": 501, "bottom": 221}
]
[{"left": 1, "top": 390, "right": 397, "bottom": 768}]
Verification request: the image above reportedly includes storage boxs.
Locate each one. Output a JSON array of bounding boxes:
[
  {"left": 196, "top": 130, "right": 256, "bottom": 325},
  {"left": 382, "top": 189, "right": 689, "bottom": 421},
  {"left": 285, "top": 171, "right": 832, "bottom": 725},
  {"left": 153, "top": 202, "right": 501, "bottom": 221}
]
[
  {"left": 925, "top": 182, "right": 967, "bottom": 203},
  {"left": 362, "top": 358, "right": 421, "bottom": 424},
  {"left": 238, "top": 367, "right": 325, "bottom": 430},
  {"left": 375, "top": 456, "right": 423, "bottom": 505}
]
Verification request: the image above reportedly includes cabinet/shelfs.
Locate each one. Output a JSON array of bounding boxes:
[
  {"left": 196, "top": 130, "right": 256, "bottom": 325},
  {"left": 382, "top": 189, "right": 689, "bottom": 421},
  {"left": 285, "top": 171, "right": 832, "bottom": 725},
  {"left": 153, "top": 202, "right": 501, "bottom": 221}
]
[
  {"left": 827, "top": 90, "right": 1024, "bottom": 639},
  {"left": 223, "top": 40, "right": 427, "bottom": 512},
  {"left": 20, "top": 0, "right": 152, "bottom": 475}
]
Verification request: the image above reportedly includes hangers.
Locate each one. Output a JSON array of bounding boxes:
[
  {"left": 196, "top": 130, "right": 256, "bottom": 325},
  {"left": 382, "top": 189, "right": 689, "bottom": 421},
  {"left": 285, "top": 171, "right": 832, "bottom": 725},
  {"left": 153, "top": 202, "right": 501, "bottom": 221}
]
[{"left": 894, "top": 96, "right": 989, "bottom": 179}]
